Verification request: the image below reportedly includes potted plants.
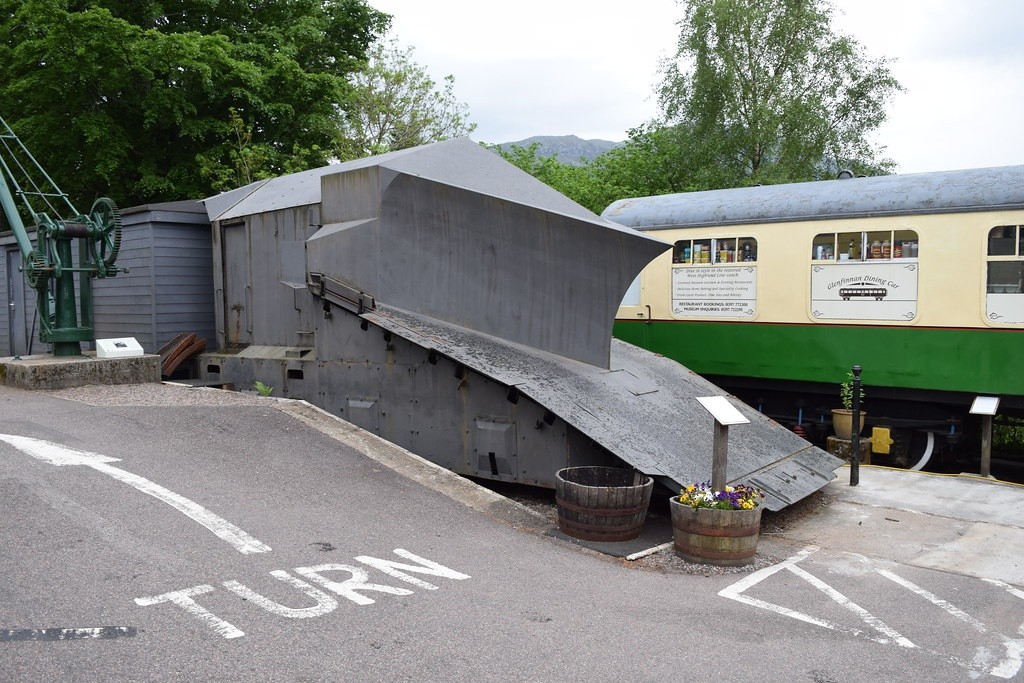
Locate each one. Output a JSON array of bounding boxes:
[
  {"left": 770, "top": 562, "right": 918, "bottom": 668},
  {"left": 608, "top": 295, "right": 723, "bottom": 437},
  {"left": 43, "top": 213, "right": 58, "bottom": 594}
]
[{"left": 831, "top": 371, "right": 866, "bottom": 439}]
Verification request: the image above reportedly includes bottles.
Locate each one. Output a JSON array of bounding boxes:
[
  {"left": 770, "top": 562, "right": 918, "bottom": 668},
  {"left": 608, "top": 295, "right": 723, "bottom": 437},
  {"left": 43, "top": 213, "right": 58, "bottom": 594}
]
[
  {"left": 821, "top": 249, "right": 826, "bottom": 260},
  {"left": 849, "top": 239, "right": 857, "bottom": 259},
  {"left": 882, "top": 240, "right": 891, "bottom": 258},
  {"left": 871, "top": 241, "right": 881, "bottom": 258},
  {"left": 911, "top": 241, "right": 918, "bottom": 257},
  {"left": 894, "top": 240, "right": 902, "bottom": 257},
  {"left": 826, "top": 246, "right": 832, "bottom": 258}
]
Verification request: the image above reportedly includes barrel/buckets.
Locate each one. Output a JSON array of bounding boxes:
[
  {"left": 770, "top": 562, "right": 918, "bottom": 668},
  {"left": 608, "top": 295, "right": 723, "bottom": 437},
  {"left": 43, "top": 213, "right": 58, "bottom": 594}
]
[{"left": 556, "top": 465, "right": 653, "bottom": 542}]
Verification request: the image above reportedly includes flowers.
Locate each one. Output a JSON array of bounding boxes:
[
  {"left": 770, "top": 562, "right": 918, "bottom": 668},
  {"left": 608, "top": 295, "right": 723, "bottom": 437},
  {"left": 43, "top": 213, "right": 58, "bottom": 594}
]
[{"left": 680, "top": 479, "right": 765, "bottom": 510}]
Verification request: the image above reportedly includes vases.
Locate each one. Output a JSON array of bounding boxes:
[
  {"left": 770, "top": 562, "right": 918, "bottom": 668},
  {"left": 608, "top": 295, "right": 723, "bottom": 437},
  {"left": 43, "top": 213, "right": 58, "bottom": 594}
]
[{"left": 670, "top": 496, "right": 762, "bottom": 567}]
